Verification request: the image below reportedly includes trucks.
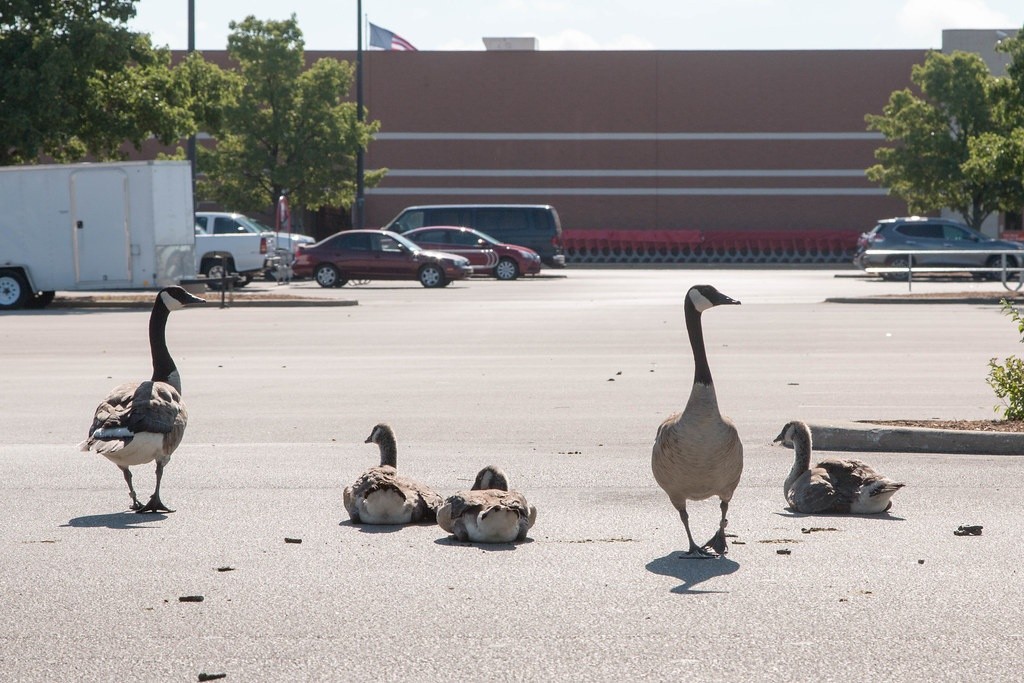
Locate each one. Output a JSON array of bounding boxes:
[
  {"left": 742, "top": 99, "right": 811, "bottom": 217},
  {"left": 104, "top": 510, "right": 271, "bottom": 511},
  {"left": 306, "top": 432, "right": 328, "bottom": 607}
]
[{"left": 0, "top": 158, "right": 197, "bottom": 310}]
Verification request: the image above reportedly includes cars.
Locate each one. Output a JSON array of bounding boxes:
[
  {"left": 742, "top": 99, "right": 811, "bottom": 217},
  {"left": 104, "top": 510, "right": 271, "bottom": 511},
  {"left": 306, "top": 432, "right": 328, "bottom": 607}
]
[
  {"left": 380, "top": 224, "right": 542, "bottom": 280},
  {"left": 289, "top": 227, "right": 474, "bottom": 289}
]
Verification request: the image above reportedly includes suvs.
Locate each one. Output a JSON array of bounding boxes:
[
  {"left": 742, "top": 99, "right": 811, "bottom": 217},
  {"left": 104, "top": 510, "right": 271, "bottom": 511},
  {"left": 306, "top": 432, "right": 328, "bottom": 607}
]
[
  {"left": 194, "top": 210, "right": 316, "bottom": 291},
  {"left": 373, "top": 201, "right": 569, "bottom": 269},
  {"left": 851, "top": 214, "right": 1024, "bottom": 283}
]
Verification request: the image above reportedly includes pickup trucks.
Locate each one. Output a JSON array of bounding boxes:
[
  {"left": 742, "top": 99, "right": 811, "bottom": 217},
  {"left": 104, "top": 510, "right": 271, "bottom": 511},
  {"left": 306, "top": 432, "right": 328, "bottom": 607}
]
[{"left": 193, "top": 224, "right": 277, "bottom": 293}]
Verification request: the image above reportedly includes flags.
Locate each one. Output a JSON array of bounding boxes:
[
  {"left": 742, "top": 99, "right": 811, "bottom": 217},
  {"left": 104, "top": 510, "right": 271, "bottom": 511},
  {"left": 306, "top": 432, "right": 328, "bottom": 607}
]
[{"left": 368, "top": 23, "right": 418, "bottom": 52}]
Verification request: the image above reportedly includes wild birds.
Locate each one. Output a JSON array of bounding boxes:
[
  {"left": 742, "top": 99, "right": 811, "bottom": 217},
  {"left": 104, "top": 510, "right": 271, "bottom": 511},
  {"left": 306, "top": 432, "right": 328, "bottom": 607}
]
[
  {"left": 652, "top": 285, "right": 745, "bottom": 559},
  {"left": 772, "top": 420, "right": 905, "bottom": 515},
  {"left": 344, "top": 424, "right": 446, "bottom": 525},
  {"left": 437, "top": 465, "right": 536, "bottom": 542},
  {"left": 78, "top": 286, "right": 206, "bottom": 514}
]
